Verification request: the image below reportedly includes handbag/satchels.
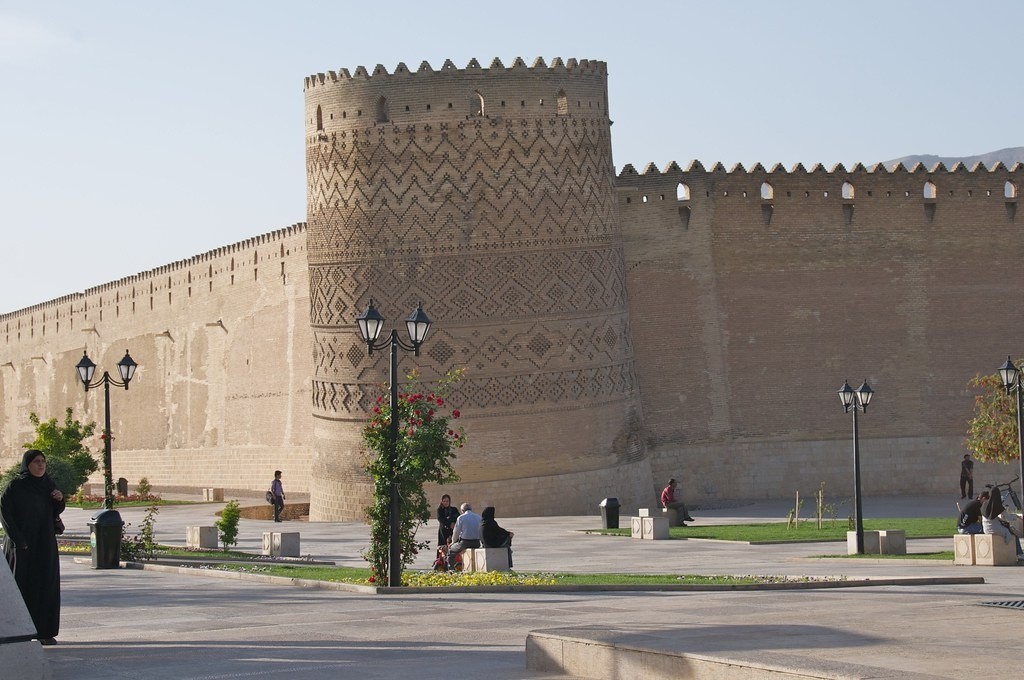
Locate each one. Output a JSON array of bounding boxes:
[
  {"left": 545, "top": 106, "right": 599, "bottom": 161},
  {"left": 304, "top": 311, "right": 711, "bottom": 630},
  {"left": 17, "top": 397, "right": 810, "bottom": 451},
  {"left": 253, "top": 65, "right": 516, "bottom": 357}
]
[
  {"left": 266, "top": 491, "right": 275, "bottom": 505},
  {"left": 1010, "top": 516, "right": 1024, "bottom": 538},
  {"left": 50, "top": 479, "right": 65, "bottom": 535}
]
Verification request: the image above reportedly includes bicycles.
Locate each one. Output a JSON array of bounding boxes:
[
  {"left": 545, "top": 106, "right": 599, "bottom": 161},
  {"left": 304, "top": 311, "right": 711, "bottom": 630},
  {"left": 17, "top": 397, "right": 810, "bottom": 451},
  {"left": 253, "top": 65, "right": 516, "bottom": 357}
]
[{"left": 985, "top": 474, "right": 1022, "bottom": 511}]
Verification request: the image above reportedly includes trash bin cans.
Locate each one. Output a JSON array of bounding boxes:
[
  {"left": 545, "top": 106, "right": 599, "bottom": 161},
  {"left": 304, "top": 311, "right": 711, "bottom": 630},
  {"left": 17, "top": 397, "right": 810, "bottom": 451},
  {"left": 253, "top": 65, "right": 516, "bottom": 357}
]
[
  {"left": 86, "top": 508, "right": 125, "bottom": 570},
  {"left": 115, "top": 477, "right": 128, "bottom": 496},
  {"left": 598, "top": 498, "right": 622, "bottom": 529}
]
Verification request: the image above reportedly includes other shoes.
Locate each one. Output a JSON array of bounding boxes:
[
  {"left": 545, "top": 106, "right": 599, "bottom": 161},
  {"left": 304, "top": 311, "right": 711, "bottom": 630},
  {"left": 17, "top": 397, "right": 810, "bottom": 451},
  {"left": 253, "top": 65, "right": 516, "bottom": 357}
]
[
  {"left": 276, "top": 519, "right": 282, "bottom": 522},
  {"left": 681, "top": 522, "right": 687, "bottom": 526},
  {"left": 38, "top": 638, "right": 57, "bottom": 645},
  {"left": 685, "top": 517, "right": 694, "bottom": 521},
  {"left": 1017, "top": 553, "right": 1024, "bottom": 560}
]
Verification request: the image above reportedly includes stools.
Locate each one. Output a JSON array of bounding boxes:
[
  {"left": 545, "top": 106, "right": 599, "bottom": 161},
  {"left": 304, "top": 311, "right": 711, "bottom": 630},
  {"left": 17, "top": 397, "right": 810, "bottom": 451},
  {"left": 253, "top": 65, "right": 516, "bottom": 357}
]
[
  {"left": 461, "top": 546, "right": 510, "bottom": 573},
  {"left": 846, "top": 529, "right": 906, "bottom": 555},
  {"left": 203, "top": 487, "right": 224, "bottom": 502},
  {"left": 630, "top": 507, "right": 681, "bottom": 540},
  {"left": 262, "top": 532, "right": 300, "bottom": 558},
  {"left": 185, "top": 526, "right": 218, "bottom": 549},
  {"left": 954, "top": 533, "right": 1017, "bottom": 566}
]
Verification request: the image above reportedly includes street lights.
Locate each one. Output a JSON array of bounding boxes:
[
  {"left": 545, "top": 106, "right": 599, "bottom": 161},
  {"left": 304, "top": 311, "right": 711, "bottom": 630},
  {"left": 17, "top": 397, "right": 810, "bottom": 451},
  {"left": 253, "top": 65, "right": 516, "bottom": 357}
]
[
  {"left": 75, "top": 349, "right": 139, "bottom": 509},
  {"left": 838, "top": 378, "right": 876, "bottom": 554},
  {"left": 355, "top": 296, "right": 435, "bottom": 586},
  {"left": 998, "top": 356, "right": 1024, "bottom": 537}
]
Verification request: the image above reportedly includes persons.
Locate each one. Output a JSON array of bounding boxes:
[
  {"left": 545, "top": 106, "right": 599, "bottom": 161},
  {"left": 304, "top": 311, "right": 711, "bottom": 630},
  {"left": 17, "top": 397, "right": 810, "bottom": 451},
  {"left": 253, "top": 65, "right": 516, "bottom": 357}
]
[
  {"left": 271, "top": 471, "right": 285, "bottom": 522},
  {"left": 436, "top": 494, "right": 482, "bottom": 575},
  {"left": 0, "top": 449, "right": 65, "bottom": 646},
  {"left": 479, "top": 507, "right": 514, "bottom": 571},
  {"left": 960, "top": 454, "right": 973, "bottom": 499},
  {"left": 661, "top": 478, "right": 695, "bottom": 527},
  {"left": 956, "top": 487, "right": 1024, "bottom": 563}
]
